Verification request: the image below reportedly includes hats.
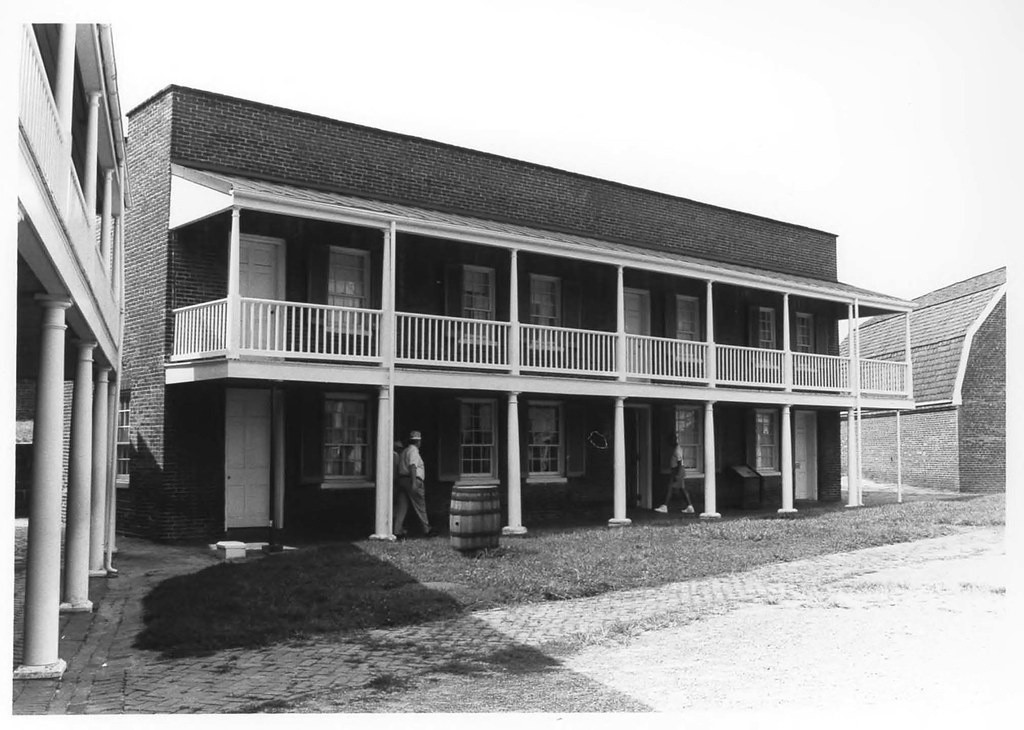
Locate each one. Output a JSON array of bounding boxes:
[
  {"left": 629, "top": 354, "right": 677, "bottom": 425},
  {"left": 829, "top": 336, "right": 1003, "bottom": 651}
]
[{"left": 410, "top": 430, "right": 422, "bottom": 440}]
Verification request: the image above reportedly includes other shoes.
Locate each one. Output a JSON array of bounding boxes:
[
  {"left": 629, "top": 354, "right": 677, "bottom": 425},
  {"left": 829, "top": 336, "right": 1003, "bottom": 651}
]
[
  {"left": 682, "top": 505, "right": 694, "bottom": 514},
  {"left": 427, "top": 531, "right": 440, "bottom": 536},
  {"left": 654, "top": 505, "right": 668, "bottom": 513},
  {"left": 395, "top": 534, "right": 407, "bottom": 539}
]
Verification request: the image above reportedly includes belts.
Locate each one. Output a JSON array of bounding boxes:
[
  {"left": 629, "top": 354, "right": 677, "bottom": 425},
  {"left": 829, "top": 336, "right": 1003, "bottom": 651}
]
[{"left": 400, "top": 475, "right": 424, "bottom": 483}]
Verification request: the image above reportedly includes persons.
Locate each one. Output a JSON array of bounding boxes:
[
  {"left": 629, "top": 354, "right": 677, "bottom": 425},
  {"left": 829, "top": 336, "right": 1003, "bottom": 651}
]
[
  {"left": 654, "top": 433, "right": 695, "bottom": 514},
  {"left": 393, "top": 431, "right": 441, "bottom": 538}
]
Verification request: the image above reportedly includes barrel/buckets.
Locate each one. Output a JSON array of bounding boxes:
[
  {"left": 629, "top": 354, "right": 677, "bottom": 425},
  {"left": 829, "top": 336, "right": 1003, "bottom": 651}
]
[{"left": 448, "top": 485, "right": 501, "bottom": 549}]
